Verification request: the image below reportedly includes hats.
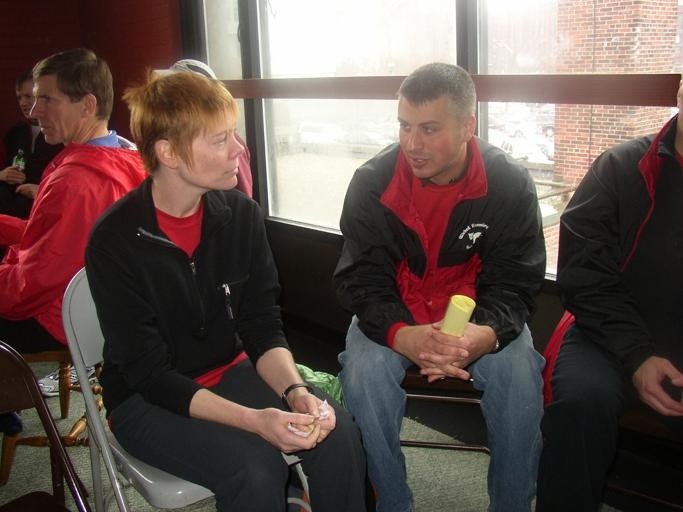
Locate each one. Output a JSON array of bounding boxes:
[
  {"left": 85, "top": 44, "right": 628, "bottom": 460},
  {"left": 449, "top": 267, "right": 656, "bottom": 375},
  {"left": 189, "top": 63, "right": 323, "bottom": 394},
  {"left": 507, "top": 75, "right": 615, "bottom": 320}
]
[{"left": 149, "top": 59, "right": 217, "bottom": 79}]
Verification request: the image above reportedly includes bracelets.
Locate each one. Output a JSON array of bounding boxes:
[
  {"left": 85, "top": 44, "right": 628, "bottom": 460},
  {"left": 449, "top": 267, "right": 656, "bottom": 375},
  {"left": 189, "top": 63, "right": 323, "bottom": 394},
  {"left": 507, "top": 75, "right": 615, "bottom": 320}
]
[{"left": 280, "top": 383, "right": 313, "bottom": 409}]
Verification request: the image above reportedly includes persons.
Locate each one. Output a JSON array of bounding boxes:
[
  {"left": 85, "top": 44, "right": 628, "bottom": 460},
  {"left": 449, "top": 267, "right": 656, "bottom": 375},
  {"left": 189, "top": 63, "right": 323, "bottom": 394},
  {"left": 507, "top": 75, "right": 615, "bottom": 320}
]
[
  {"left": 0, "top": 70, "right": 65, "bottom": 259},
  {"left": 83, "top": 67, "right": 368, "bottom": 512},
  {"left": 535, "top": 74, "right": 682, "bottom": 512},
  {"left": 173, "top": 57, "right": 252, "bottom": 201},
  {"left": 331, "top": 60, "right": 546, "bottom": 512},
  {"left": 0, "top": 42, "right": 150, "bottom": 437}
]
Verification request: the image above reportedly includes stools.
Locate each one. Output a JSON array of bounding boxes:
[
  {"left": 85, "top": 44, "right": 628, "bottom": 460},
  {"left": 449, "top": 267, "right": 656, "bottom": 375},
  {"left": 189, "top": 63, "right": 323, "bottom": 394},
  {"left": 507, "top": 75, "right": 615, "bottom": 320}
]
[
  {"left": 600, "top": 403, "right": 681, "bottom": 510},
  {"left": 394, "top": 362, "right": 495, "bottom": 455}
]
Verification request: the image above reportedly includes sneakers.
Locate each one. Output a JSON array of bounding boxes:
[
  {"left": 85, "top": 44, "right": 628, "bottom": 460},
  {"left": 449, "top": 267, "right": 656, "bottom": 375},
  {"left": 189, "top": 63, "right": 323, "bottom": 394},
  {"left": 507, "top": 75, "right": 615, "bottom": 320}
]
[
  {"left": 0, "top": 411, "right": 23, "bottom": 435},
  {"left": 37, "top": 362, "right": 98, "bottom": 396}
]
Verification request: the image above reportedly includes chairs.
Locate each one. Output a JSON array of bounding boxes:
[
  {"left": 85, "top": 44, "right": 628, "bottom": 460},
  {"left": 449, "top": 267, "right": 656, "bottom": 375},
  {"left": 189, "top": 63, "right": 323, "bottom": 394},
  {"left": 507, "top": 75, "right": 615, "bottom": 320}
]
[
  {"left": 0, "top": 336, "right": 105, "bottom": 512},
  {"left": 58, "top": 265, "right": 313, "bottom": 512}
]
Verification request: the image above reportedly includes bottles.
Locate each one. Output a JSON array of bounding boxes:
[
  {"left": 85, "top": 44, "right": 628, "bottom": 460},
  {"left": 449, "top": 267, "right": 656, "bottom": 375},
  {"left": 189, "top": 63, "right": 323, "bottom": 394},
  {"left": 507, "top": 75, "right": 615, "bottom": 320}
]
[{"left": 7, "top": 148, "right": 25, "bottom": 187}]
